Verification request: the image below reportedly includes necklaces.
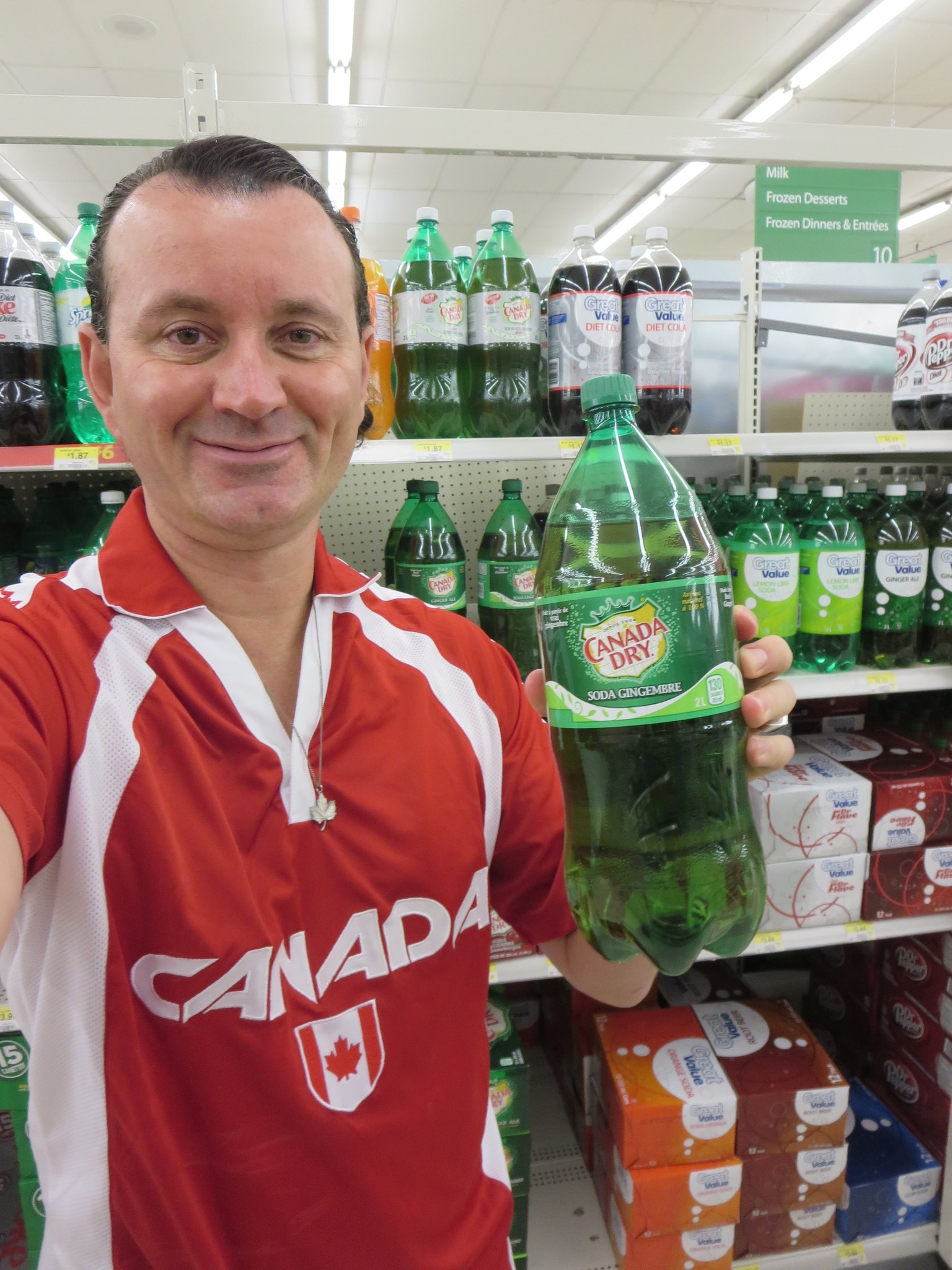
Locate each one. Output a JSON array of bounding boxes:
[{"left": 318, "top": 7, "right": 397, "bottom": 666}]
[{"left": 268, "top": 588, "right": 336, "bottom": 831}]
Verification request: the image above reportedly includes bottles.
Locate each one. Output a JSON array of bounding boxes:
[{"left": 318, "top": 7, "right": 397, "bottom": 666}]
[
  {"left": 530, "top": 485, "right": 561, "bottom": 537},
  {"left": 0, "top": 199, "right": 82, "bottom": 448},
  {"left": 390, "top": 206, "right": 467, "bottom": 441},
  {"left": 532, "top": 374, "right": 766, "bottom": 976},
  {"left": 454, "top": 245, "right": 473, "bottom": 286},
  {"left": 83, "top": 491, "right": 124, "bottom": 559},
  {"left": 890, "top": 271, "right": 945, "bottom": 430},
  {"left": 467, "top": 209, "right": 540, "bottom": 437},
  {"left": 52, "top": 203, "right": 118, "bottom": 444},
  {"left": 0, "top": 483, "right": 86, "bottom": 592},
  {"left": 729, "top": 486, "right": 799, "bottom": 656},
  {"left": 538, "top": 223, "right": 623, "bottom": 437},
  {"left": 793, "top": 485, "right": 865, "bottom": 674},
  {"left": 337, "top": 206, "right": 395, "bottom": 441},
  {"left": 858, "top": 485, "right": 930, "bottom": 668},
  {"left": 920, "top": 286, "right": 952, "bottom": 430},
  {"left": 685, "top": 476, "right": 771, "bottom": 564},
  {"left": 774, "top": 464, "right": 952, "bottom": 531},
  {"left": 918, "top": 484, "right": 952, "bottom": 667},
  {"left": 618, "top": 226, "right": 693, "bottom": 436},
  {"left": 383, "top": 481, "right": 465, "bottom": 621},
  {"left": 476, "top": 479, "right": 544, "bottom": 684},
  {"left": 588, "top": 485, "right": 631, "bottom": 512}
]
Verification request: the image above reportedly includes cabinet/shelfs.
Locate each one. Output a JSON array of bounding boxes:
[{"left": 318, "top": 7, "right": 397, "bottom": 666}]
[{"left": 0, "top": 248, "right": 951, "bottom": 1270}]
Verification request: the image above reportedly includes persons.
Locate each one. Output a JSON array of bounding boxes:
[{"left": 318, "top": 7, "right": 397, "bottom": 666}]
[{"left": 0, "top": 135, "right": 796, "bottom": 1270}]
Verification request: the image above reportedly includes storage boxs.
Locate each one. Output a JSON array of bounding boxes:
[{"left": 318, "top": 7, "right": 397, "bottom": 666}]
[{"left": 487, "top": 693, "right": 951, "bottom": 1270}]
